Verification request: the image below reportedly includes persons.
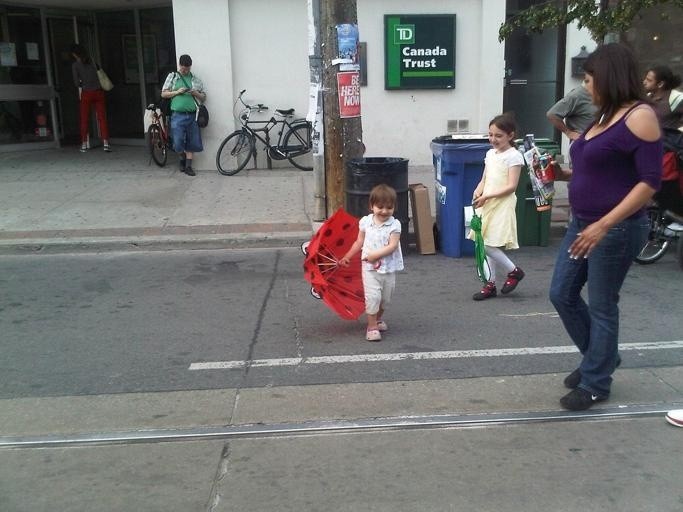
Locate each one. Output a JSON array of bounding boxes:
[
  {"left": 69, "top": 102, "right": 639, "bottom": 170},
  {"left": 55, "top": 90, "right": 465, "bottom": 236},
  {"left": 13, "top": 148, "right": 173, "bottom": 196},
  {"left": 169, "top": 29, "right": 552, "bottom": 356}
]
[
  {"left": 642, "top": 64, "right": 683, "bottom": 240},
  {"left": 531, "top": 153, "right": 543, "bottom": 179},
  {"left": 72, "top": 43, "right": 114, "bottom": 152},
  {"left": 343, "top": 182, "right": 405, "bottom": 342},
  {"left": 665, "top": 408, "right": 683, "bottom": 428},
  {"left": 161, "top": 54, "right": 207, "bottom": 177},
  {"left": 546, "top": 72, "right": 603, "bottom": 143},
  {"left": 471, "top": 113, "right": 526, "bottom": 302},
  {"left": 547, "top": 40, "right": 663, "bottom": 411}
]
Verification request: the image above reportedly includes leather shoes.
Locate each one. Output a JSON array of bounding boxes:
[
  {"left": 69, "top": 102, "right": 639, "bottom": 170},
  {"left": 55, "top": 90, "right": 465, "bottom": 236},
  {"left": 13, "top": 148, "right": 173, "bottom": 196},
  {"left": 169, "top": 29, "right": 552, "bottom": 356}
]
[{"left": 179, "top": 159, "right": 196, "bottom": 176}]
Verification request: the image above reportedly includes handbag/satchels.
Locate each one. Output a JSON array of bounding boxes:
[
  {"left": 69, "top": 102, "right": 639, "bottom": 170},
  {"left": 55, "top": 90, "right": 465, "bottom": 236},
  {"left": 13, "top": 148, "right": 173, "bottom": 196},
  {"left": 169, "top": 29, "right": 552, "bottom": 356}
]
[
  {"left": 197, "top": 104, "right": 209, "bottom": 128},
  {"left": 92, "top": 59, "right": 114, "bottom": 92}
]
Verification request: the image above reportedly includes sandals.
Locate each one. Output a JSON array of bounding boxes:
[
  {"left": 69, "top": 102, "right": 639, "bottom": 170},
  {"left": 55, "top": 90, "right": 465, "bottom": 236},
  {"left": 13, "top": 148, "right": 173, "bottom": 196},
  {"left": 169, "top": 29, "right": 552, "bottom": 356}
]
[
  {"left": 366, "top": 323, "right": 381, "bottom": 341},
  {"left": 377, "top": 318, "right": 388, "bottom": 331}
]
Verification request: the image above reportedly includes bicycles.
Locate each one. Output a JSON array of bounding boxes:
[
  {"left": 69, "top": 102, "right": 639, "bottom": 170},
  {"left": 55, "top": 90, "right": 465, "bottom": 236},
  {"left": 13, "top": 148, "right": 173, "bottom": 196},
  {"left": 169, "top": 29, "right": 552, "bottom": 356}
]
[
  {"left": 143, "top": 99, "right": 176, "bottom": 170},
  {"left": 214, "top": 89, "right": 314, "bottom": 176},
  {"left": 631, "top": 206, "right": 683, "bottom": 266}
]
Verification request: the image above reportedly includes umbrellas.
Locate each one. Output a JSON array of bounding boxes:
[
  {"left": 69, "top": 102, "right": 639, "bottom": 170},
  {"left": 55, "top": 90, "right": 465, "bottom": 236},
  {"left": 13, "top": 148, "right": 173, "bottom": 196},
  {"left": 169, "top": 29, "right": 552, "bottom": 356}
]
[
  {"left": 300, "top": 204, "right": 367, "bottom": 324},
  {"left": 469, "top": 205, "right": 493, "bottom": 290}
]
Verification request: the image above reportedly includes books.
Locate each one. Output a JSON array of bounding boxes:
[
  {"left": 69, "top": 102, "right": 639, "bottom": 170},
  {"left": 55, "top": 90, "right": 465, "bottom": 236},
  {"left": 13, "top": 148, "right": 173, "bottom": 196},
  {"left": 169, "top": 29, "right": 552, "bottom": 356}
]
[{"left": 523, "top": 147, "right": 554, "bottom": 199}]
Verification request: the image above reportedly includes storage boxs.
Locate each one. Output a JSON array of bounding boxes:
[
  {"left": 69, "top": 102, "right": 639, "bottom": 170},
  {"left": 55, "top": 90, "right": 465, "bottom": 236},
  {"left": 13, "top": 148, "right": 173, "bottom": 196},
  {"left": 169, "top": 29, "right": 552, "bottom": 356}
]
[{"left": 409, "top": 181, "right": 436, "bottom": 255}]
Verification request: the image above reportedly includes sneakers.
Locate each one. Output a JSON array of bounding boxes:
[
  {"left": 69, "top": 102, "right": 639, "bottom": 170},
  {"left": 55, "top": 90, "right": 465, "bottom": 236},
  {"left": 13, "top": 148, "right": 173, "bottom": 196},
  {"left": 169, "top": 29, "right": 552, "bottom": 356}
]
[
  {"left": 80, "top": 145, "right": 113, "bottom": 154},
  {"left": 560, "top": 355, "right": 622, "bottom": 411},
  {"left": 501, "top": 266, "right": 525, "bottom": 294},
  {"left": 665, "top": 408, "right": 682, "bottom": 426},
  {"left": 472, "top": 282, "right": 497, "bottom": 301}
]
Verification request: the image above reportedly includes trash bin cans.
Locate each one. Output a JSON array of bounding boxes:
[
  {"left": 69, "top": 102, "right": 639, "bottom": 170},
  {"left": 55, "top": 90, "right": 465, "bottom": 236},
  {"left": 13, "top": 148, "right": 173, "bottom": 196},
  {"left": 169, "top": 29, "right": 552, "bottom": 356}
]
[
  {"left": 514, "top": 139, "right": 562, "bottom": 247},
  {"left": 429, "top": 135, "right": 513, "bottom": 259}
]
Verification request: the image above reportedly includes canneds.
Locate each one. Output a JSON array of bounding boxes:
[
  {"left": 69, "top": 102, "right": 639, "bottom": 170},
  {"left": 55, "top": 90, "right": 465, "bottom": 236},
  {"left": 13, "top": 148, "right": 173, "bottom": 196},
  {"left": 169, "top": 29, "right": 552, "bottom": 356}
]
[{"left": 536, "top": 153, "right": 556, "bottom": 184}]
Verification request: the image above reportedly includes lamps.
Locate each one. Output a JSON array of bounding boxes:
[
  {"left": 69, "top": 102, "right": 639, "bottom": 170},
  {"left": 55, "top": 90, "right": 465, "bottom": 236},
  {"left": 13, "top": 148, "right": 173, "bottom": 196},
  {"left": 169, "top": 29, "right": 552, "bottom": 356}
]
[{"left": 571, "top": 46, "right": 589, "bottom": 75}]
